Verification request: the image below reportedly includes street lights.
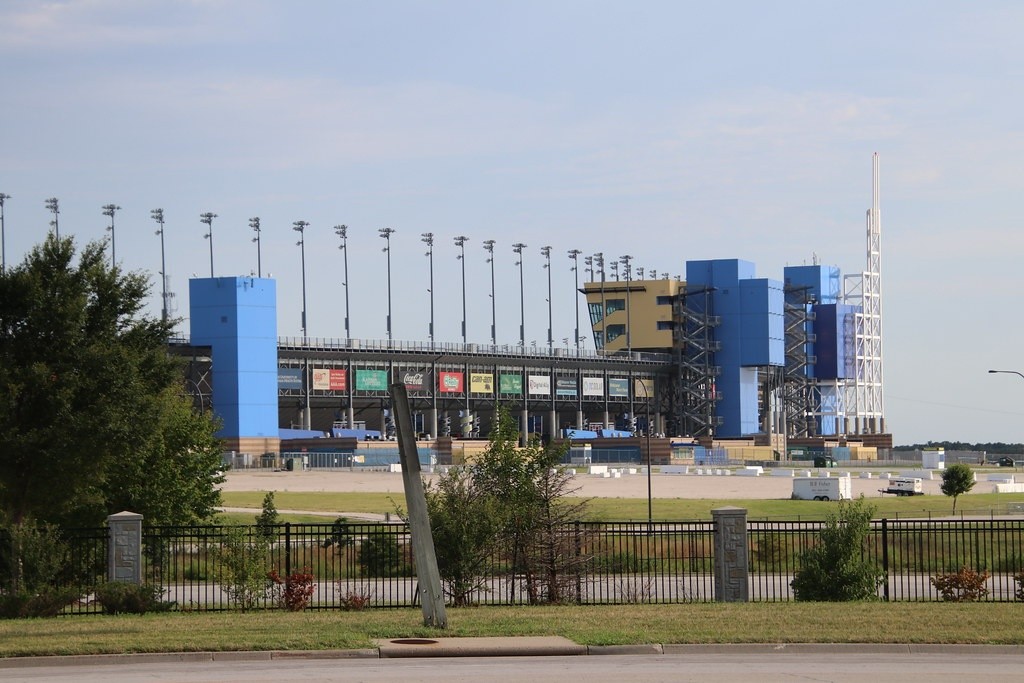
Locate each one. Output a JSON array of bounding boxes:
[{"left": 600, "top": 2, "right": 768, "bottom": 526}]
[
  {"left": 568, "top": 249, "right": 581, "bottom": 358},
  {"left": 293, "top": 220, "right": 310, "bottom": 346},
  {"left": 201, "top": 213, "right": 218, "bottom": 278},
  {"left": 378, "top": 227, "right": 394, "bottom": 349},
  {"left": 44, "top": 196, "right": 60, "bottom": 245},
  {"left": 151, "top": 207, "right": 168, "bottom": 321},
  {"left": 249, "top": 216, "right": 261, "bottom": 277},
  {"left": 334, "top": 224, "right": 350, "bottom": 345},
  {"left": 512, "top": 242, "right": 527, "bottom": 354},
  {"left": 629, "top": 375, "right": 651, "bottom": 533},
  {"left": 482, "top": 239, "right": 497, "bottom": 353},
  {"left": 422, "top": 232, "right": 436, "bottom": 348},
  {"left": 0, "top": 192, "right": 12, "bottom": 270},
  {"left": 102, "top": 204, "right": 121, "bottom": 269},
  {"left": 543, "top": 245, "right": 556, "bottom": 355},
  {"left": 584, "top": 253, "right": 681, "bottom": 359},
  {"left": 453, "top": 235, "right": 470, "bottom": 351}
]
